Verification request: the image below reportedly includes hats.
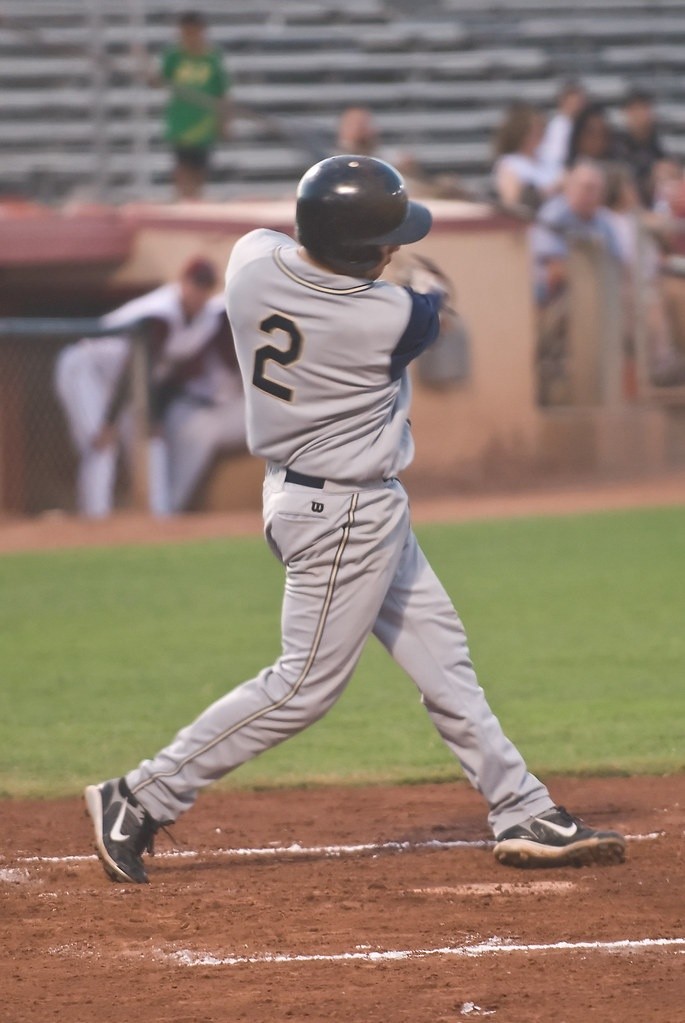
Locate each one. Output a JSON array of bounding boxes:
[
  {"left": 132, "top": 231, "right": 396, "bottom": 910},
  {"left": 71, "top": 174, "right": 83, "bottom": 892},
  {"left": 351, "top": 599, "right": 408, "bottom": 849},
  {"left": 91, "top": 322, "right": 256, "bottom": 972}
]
[{"left": 185, "top": 257, "right": 213, "bottom": 287}]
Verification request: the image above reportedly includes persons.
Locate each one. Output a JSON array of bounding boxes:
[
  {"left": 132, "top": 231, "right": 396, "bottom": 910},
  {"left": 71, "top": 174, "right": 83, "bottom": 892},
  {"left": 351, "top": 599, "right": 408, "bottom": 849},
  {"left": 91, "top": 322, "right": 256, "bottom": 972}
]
[
  {"left": 320, "top": 106, "right": 407, "bottom": 167},
  {"left": 85, "top": 154, "right": 624, "bottom": 882},
  {"left": 56, "top": 254, "right": 247, "bottom": 526},
  {"left": 148, "top": 10, "right": 230, "bottom": 198},
  {"left": 494, "top": 83, "right": 685, "bottom": 399}
]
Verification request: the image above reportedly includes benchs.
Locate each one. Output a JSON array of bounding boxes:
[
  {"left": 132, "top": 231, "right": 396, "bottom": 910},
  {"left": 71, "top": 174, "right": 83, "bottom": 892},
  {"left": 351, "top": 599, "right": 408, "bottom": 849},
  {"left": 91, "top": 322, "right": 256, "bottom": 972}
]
[{"left": 0, "top": 0, "right": 685, "bottom": 204}]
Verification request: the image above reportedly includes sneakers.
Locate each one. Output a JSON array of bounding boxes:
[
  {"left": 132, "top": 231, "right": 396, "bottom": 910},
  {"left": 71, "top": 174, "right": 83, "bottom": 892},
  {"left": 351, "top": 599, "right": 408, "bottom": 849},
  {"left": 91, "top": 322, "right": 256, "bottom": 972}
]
[
  {"left": 80, "top": 778, "right": 177, "bottom": 884},
  {"left": 492, "top": 806, "right": 626, "bottom": 869}
]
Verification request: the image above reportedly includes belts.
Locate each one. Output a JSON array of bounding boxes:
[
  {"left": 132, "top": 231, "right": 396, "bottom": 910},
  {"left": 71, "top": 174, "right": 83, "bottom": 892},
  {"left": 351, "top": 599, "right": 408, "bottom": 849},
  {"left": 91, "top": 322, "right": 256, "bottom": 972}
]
[{"left": 285, "top": 467, "right": 325, "bottom": 490}]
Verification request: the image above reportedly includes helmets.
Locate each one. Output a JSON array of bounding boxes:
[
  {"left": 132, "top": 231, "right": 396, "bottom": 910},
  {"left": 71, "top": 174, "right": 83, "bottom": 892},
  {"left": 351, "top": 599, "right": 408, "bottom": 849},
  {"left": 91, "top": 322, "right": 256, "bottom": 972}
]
[{"left": 295, "top": 155, "right": 432, "bottom": 275}]
